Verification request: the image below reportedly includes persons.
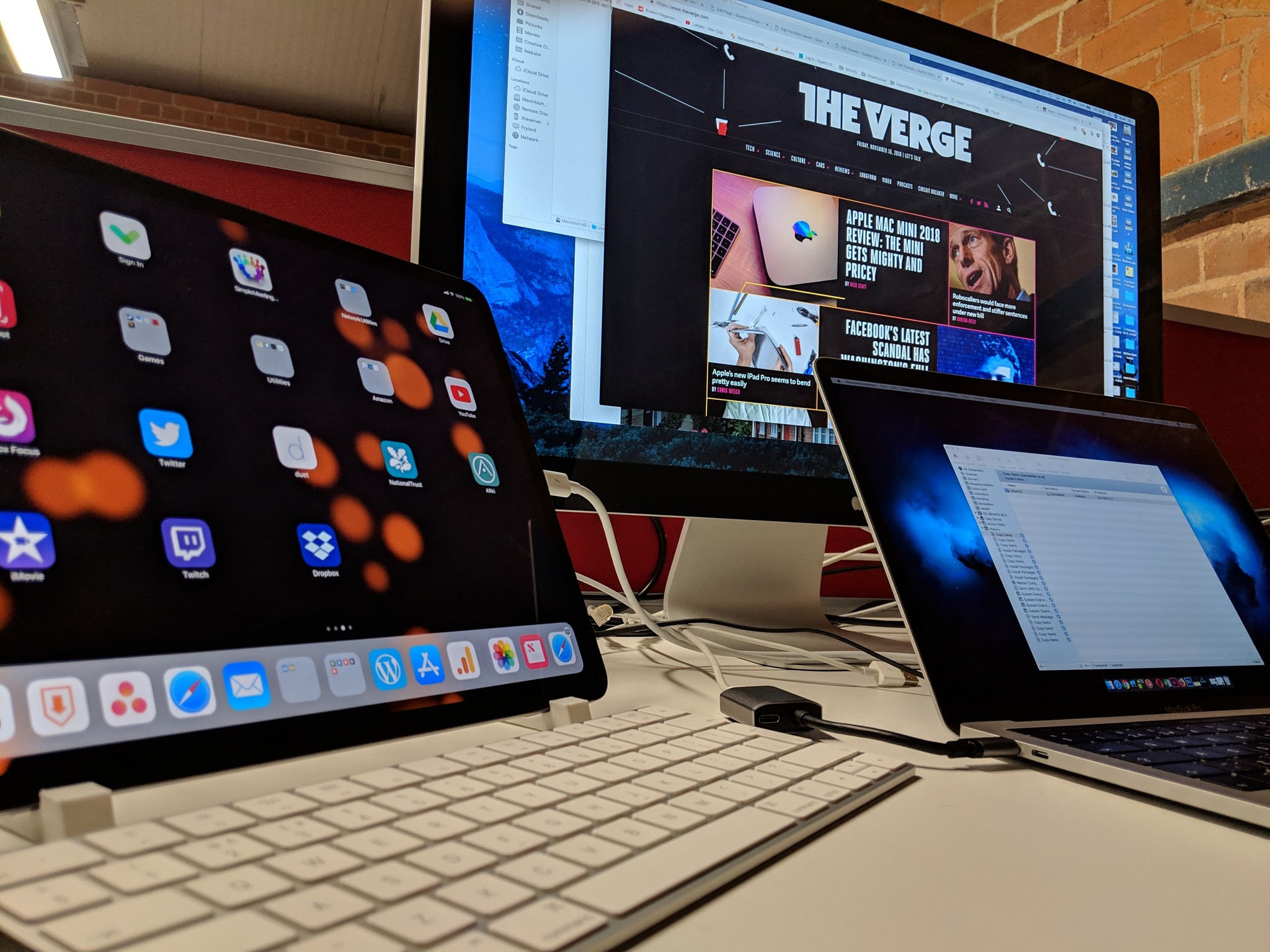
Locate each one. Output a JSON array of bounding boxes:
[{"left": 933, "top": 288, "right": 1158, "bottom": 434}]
[
  {"left": 726, "top": 322, "right": 794, "bottom": 374},
  {"left": 947, "top": 221, "right": 1034, "bottom": 304}
]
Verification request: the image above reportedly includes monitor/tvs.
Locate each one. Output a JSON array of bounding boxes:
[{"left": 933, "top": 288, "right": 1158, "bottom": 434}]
[{"left": 410, "top": 0, "right": 1162, "bottom": 664}]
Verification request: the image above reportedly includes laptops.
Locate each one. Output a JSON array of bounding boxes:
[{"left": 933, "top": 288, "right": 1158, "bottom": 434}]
[
  {"left": 808, "top": 357, "right": 1270, "bottom": 831},
  {"left": 0, "top": 125, "right": 921, "bottom": 952}
]
